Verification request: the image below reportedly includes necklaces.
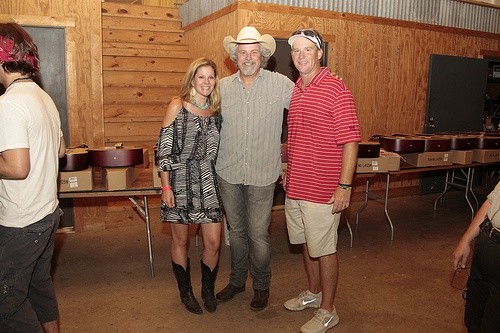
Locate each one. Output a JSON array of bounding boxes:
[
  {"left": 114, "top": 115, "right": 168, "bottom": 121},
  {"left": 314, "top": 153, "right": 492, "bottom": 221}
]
[
  {"left": 7, "top": 77, "right": 32, "bottom": 88},
  {"left": 191, "top": 97, "right": 209, "bottom": 110}
]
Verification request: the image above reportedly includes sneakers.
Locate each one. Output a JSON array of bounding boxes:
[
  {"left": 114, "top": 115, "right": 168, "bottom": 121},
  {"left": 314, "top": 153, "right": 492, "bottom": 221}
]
[
  {"left": 250, "top": 288, "right": 269, "bottom": 310},
  {"left": 284, "top": 289, "right": 322, "bottom": 311},
  {"left": 216, "top": 284, "right": 244, "bottom": 302},
  {"left": 299, "top": 305, "right": 339, "bottom": 333}
]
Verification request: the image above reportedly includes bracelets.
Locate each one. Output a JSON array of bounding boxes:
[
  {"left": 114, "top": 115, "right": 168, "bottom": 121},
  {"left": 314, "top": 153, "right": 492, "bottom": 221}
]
[
  {"left": 161, "top": 185, "right": 172, "bottom": 192},
  {"left": 338, "top": 182, "right": 353, "bottom": 190}
]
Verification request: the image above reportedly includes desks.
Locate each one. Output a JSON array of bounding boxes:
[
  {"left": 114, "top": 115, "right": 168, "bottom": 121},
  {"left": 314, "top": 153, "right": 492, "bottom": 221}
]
[
  {"left": 344, "top": 161, "right": 500, "bottom": 247},
  {"left": 57, "top": 169, "right": 163, "bottom": 278}
]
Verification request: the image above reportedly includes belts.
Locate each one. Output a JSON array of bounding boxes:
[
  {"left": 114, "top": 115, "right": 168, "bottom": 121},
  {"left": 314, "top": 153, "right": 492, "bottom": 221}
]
[{"left": 484, "top": 221, "right": 500, "bottom": 243}]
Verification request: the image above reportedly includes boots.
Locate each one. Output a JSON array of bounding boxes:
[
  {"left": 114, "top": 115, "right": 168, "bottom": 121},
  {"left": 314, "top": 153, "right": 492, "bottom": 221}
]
[
  {"left": 172, "top": 257, "right": 201, "bottom": 314},
  {"left": 200, "top": 258, "right": 218, "bottom": 312}
]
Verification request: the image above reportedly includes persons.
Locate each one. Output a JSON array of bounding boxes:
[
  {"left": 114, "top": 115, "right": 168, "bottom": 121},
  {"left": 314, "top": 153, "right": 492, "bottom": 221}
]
[
  {"left": 216, "top": 27, "right": 343, "bottom": 311},
  {"left": 284, "top": 30, "right": 362, "bottom": 333},
  {"left": 451, "top": 180, "right": 500, "bottom": 333},
  {"left": 156, "top": 58, "right": 224, "bottom": 314},
  {"left": 0, "top": 22, "right": 66, "bottom": 333}
]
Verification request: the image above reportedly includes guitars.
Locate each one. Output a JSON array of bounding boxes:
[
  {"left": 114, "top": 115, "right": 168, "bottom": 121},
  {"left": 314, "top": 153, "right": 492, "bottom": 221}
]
[
  {"left": 367, "top": 131, "right": 500, "bottom": 153},
  {"left": 58, "top": 143, "right": 89, "bottom": 172},
  {"left": 89, "top": 142, "right": 143, "bottom": 168}
]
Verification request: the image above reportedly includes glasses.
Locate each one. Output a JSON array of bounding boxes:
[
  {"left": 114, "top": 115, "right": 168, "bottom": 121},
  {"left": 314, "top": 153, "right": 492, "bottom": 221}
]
[{"left": 292, "top": 30, "right": 321, "bottom": 48}]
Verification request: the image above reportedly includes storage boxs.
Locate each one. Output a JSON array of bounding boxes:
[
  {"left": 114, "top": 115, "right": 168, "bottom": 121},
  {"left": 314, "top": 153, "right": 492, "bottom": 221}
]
[
  {"left": 58, "top": 149, "right": 163, "bottom": 192},
  {"left": 355, "top": 150, "right": 500, "bottom": 173}
]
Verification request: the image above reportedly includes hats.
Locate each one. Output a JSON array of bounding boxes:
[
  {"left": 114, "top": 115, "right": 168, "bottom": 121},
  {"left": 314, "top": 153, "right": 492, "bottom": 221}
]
[
  {"left": 223, "top": 27, "right": 276, "bottom": 59},
  {"left": 288, "top": 30, "right": 321, "bottom": 49}
]
